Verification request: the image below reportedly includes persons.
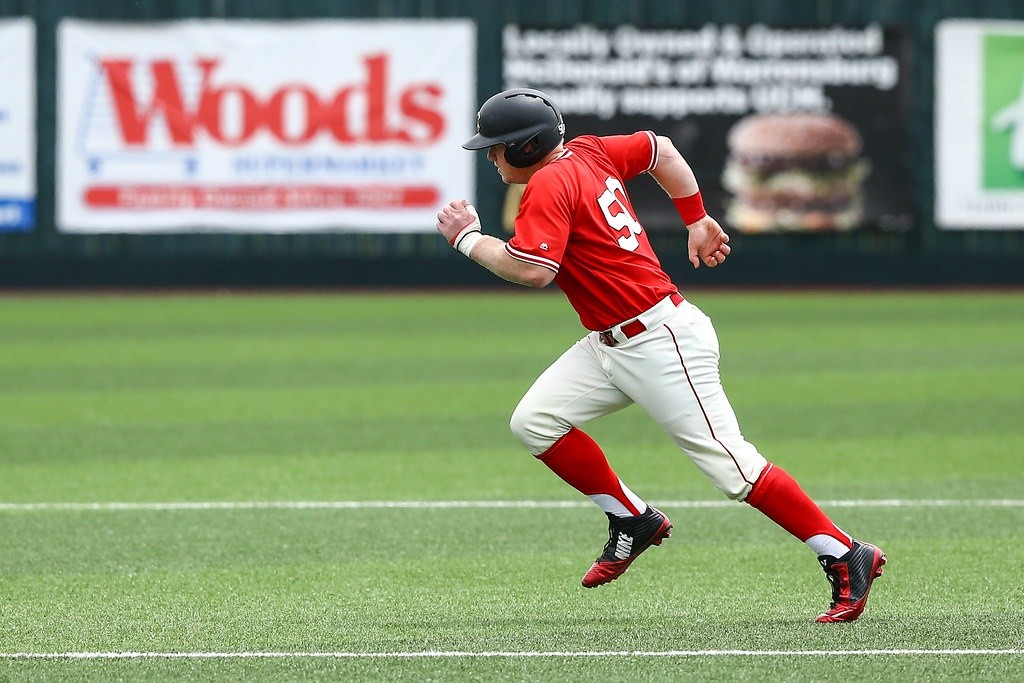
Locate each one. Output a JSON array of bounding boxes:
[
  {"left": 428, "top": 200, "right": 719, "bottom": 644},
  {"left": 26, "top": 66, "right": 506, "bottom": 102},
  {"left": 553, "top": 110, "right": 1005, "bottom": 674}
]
[{"left": 436, "top": 87, "right": 888, "bottom": 623}]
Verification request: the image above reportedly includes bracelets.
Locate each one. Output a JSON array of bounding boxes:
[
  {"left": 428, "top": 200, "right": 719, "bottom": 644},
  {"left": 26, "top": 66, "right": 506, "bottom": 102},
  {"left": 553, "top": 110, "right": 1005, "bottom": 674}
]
[{"left": 672, "top": 190, "right": 707, "bottom": 227}]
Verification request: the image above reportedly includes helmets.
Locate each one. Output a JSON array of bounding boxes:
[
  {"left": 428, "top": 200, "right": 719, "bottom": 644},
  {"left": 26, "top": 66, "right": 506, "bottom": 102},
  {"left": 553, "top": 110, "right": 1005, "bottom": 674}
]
[{"left": 462, "top": 88, "right": 566, "bottom": 169}]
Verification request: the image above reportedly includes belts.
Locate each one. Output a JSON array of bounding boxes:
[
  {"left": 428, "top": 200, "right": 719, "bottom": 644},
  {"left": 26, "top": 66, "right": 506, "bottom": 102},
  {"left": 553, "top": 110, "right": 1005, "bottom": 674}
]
[{"left": 599, "top": 293, "right": 684, "bottom": 347}]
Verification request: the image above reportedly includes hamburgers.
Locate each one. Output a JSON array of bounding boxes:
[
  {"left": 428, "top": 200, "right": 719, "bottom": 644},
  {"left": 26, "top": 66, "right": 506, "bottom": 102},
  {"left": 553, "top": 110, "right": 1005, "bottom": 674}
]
[{"left": 722, "top": 111, "right": 864, "bottom": 234}]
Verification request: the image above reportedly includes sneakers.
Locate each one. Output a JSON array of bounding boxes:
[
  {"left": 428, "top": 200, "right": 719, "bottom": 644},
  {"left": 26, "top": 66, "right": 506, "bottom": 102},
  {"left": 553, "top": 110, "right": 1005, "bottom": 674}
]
[
  {"left": 815, "top": 538, "right": 887, "bottom": 622},
  {"left": 582, "top": 505, "right": 673, "bottom": 589}
]
